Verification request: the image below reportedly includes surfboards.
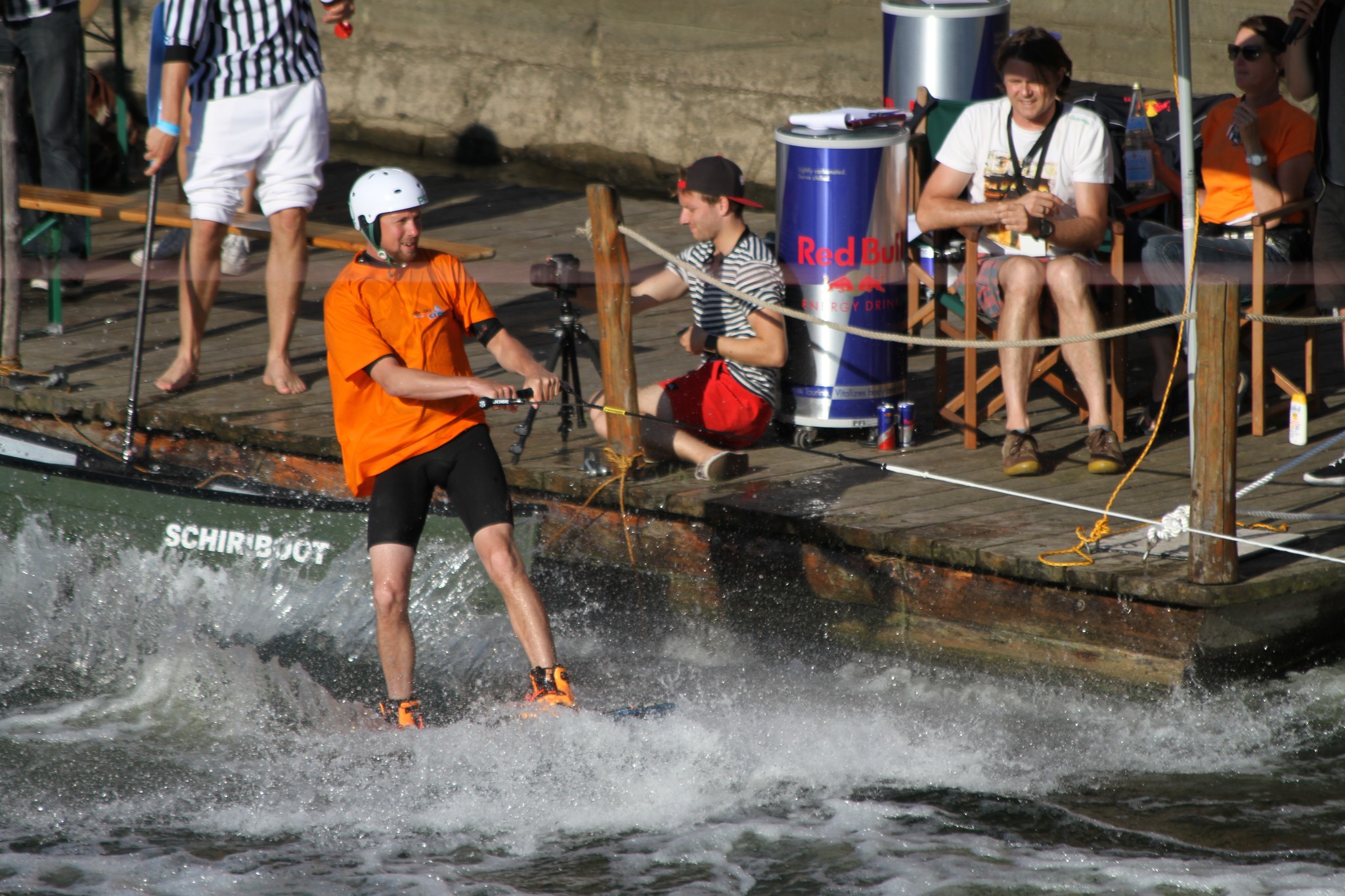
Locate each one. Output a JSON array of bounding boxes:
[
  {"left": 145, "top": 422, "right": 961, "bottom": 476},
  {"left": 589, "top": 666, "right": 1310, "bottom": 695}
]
[{"left": 376, "top": 681, "right": 677, "bottom": 733}]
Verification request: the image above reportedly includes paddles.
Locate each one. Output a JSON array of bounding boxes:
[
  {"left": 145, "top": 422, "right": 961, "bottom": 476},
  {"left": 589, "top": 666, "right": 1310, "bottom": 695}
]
[{"left": 120, "top": 1, "right": 168, "bottom": 461}]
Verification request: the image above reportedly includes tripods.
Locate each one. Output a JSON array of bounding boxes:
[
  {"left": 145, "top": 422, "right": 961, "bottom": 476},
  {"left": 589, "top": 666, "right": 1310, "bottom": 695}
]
[{"left": 509, "top": 287, "right": 601, "bottom": 466}]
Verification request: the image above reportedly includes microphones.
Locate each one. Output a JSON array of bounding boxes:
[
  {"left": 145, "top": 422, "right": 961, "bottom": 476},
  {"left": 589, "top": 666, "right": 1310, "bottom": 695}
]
[{"left": 1282, "top": 0, "right": 1319, "bottom": 44}]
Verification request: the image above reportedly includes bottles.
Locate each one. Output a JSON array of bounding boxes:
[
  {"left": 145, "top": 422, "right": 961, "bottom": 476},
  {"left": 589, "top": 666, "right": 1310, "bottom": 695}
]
[{"left": 1124, "top": 81, "right": 1155, "bottom": 190}]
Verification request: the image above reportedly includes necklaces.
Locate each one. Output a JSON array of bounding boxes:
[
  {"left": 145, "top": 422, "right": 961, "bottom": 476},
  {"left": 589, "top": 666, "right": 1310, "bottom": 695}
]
[{"left": 1007, "top": 99, "right": 1064, "bottom": 197}]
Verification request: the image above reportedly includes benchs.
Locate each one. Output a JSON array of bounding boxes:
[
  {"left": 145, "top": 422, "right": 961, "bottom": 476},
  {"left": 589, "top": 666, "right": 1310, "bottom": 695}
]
[{"left": 17, "top": 185, "right": 497, "bottom": 337}]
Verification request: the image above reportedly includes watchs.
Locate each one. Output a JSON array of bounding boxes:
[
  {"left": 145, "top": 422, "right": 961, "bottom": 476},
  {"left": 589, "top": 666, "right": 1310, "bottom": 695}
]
[
  {"left": 1245, "top": 154, "right": 1268, "bottom": 165},
  {"left": 1034, "top": 217, "right": 1055, "bottom": 240},
  {"left": 702, "top": 332, "right": 722, "bottom": 360}
]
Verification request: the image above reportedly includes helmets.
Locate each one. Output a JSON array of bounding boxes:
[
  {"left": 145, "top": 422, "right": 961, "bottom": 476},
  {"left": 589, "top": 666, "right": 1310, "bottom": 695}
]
[{"left": 348, "top": 167, "right": 429, "bottom": 231}]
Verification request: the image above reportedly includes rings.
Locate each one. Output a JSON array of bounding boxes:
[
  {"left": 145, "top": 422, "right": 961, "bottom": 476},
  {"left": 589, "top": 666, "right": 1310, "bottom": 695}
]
[{"left": 1042, "top": 207, "right": 1046, "bottom": 214}]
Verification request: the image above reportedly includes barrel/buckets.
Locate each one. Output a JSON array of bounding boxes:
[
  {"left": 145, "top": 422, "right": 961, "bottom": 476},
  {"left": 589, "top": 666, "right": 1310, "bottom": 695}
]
[
  {"left": 773, "top": 124, "right": 912, "bottom": 428},
  {"left": 880, "top": 1, "right": 1010, "bottom": 136}
]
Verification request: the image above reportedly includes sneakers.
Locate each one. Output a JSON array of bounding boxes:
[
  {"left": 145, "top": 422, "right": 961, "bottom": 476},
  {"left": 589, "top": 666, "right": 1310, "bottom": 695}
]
[
  {"left": 522, "top": 663, "right": 579, "bottom": 717},
  {"left": 130, "top": 227, "right": 190, "bottom": 269},
  {"left": 1001, "top": 427, "right": 1040, "bottom": 475},
  {"left": 1303, "top": 455, "right": 1345, "bottom": 486},
  {"left": 219, "top": 234, "right": 250, "bottom": 277},
  {"left": 379, "top": 697, "right": 424, "bottom": 732},
  {"left": 1084, "top": 428, "right": 1126, "bottom": 473}
]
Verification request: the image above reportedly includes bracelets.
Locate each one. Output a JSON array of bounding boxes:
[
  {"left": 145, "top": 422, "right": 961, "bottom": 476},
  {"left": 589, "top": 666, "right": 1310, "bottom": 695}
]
[
  {"left": 1293, "top": 23, "right": 1313, "bottom": 46},
  {"left": 154, "top": 120, "right": 180, "bottom": 136}
]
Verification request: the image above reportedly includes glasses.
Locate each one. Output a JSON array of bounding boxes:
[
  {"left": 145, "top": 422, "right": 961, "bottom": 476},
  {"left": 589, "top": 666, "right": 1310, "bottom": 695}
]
[{"left": 1227, "top": 44, "right": 1270, "bottom": 61}]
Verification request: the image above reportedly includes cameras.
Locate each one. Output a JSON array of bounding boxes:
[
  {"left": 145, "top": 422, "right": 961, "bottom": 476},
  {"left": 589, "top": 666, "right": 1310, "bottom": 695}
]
[
  {"left": 933, "top": 240, "right": 966, "bottom": 263},
  {"left": 530, "top": 254, "right": 581, "bottom": 291}
]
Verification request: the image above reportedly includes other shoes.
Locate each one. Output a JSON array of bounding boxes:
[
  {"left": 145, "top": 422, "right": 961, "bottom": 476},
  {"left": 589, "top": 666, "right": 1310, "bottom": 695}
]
[{"left": 694, "top": 451, "right": 749, "bottom": 482}]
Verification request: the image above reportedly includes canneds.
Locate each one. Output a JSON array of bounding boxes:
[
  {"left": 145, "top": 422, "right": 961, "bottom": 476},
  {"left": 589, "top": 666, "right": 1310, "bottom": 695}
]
[
  {"left": 771, "top": 120, "right": 912, "bottom": 429},
  {"left": 877, "top": 402, "right": 894, "bottom": 450},
  {"left": 897, "top": 401, "right": 916, "bottom": 447},
  {"left": 880, "top": 0, "right": 1015, "bottom": 125}
]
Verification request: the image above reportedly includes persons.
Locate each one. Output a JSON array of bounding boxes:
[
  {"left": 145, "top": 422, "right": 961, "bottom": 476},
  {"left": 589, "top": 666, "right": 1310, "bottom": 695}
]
[
  {"left": 916, "top": 26, "right": 1126, "bottom": 477},
  {"left": 323, "top": 165, "right": 577, "bottom": 728},
  {"left": 144, "top": 0, "right": 356, "bottom": 395},
  {"left": 546, "top": 155, "right": 790, "bottom": 482},
  {"left": 129, "top": 0, "right": 258, "bottom": 276},
  {"left": 1284, "top": 0, "right": 1345, "bottom": 488},
  {"left": 1123, "top": 15, "right": 1316, "bottom": 435},
  {"left": 0, "top": 0, "right": 100, "bottom": 294}
]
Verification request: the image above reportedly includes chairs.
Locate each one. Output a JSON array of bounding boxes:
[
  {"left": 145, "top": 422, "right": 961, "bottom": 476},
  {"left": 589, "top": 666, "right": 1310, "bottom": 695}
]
[{"left": 905, "top": 87, "right": 1328, "bottom": 452}]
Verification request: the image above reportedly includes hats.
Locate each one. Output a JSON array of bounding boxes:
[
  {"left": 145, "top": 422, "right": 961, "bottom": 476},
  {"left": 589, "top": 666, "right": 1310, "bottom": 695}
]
[{"left": 677, "top": 154, "right": 763, "bottom": 208}]
